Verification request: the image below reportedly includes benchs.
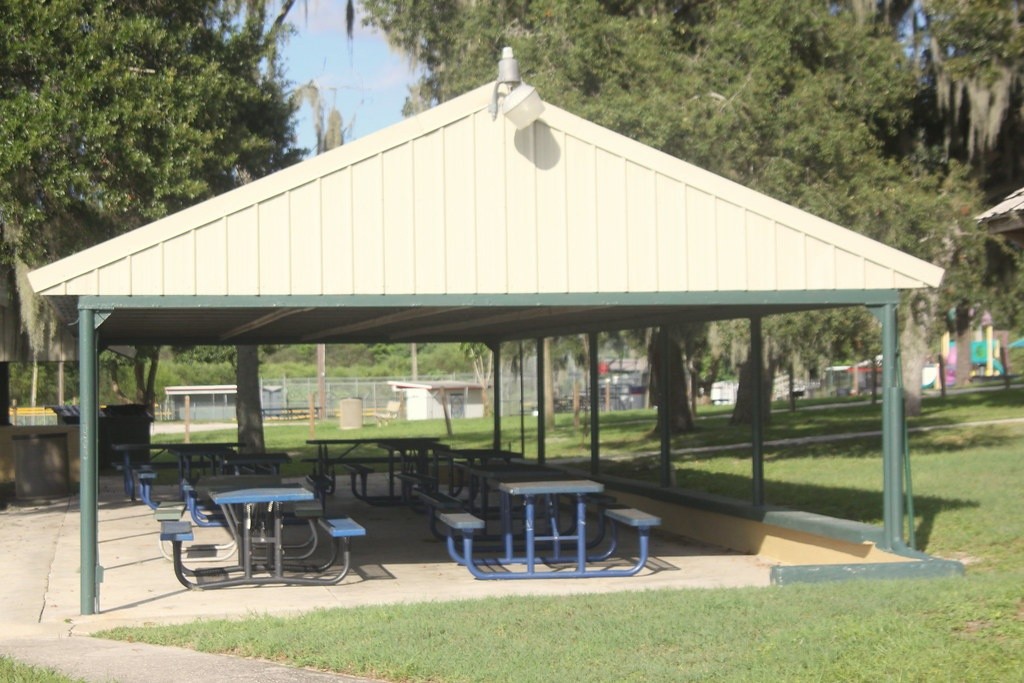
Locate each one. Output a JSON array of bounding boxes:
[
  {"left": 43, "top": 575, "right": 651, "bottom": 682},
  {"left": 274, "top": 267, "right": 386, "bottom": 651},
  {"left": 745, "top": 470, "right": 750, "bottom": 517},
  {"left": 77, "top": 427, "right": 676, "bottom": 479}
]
[
  {"left": 375, "top": 399, "right": 402, "bottom": 427},
  {"left": 262, "top": 407, "right": 322, "bottom": 421},
  {"left": 513, "top": 458, "right": 884, "bottom": 565},
  {"left": 110, "top": 455, "right": 662, "bottom": 591}
]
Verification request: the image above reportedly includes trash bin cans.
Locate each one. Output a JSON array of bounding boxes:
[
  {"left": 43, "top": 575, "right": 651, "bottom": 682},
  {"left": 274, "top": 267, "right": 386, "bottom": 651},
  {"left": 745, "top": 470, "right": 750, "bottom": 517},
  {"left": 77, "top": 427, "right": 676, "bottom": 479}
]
[
  {"left": 52, "top": 404, "right": 155, "bottom": 469},
  {"left": 11, "top": 432, "right": 70, "bottom": 501},
  {"left": 339, "top": 396, "right": 363, "bottom": 430}
]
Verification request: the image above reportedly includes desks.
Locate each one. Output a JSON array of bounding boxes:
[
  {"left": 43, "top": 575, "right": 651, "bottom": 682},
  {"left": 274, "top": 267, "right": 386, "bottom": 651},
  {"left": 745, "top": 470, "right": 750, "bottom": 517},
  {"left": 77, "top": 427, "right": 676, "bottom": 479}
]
[
  {"left": 111, "top": 442, "right": 247, "bottom": 496},
  {"left": 454, "top": 461, "right": 556, "bottom": 517},
  {"left": 168, "top": 448, "right": 237, "bottom": 505},
  {"left": 222, "top": 453, "right": 292, "bottom": 528},
  {"left": 486, "top": 473, "right": 604, "bottom": 580},
  {"left": 436, "top": 449, "right": 524, "bottom": 513},
  {"left": 377, "top": 440, "right": 450, "bottom": 505},
  {"left": 305, "top": 438, "right": 442, "bottom": 493},
  {"left": 199, "top": 474, "right": 314, "bottom": 590}
]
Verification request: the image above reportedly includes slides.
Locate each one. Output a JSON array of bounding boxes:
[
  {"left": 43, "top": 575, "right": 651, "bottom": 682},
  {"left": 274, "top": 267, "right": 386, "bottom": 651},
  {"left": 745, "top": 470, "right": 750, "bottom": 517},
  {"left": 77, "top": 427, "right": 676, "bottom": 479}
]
[{"left": 991, "top": 357, "right": 1005, "bottom": 377}]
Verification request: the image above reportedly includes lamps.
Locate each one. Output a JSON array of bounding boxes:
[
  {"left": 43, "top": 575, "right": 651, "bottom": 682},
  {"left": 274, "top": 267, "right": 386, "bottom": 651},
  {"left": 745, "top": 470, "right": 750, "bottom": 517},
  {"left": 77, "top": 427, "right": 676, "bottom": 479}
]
[{"left": 489, "top": 46, "right": 546, "bottom": 130}]
